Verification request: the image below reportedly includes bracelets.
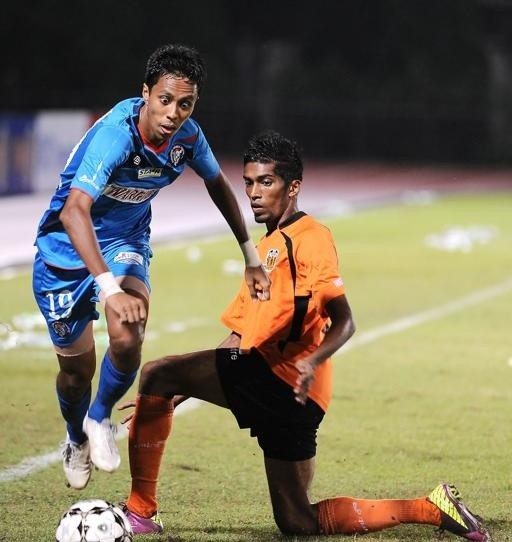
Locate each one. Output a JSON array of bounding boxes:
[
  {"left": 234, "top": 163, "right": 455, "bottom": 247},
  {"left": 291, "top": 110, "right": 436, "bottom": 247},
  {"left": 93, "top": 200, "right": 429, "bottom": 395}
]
[
  {"left": 96, "top": 271, "right": 126, "bottom": 298},
  {"left": 239, "top": 239, "right": 261, "bottom": 267}
]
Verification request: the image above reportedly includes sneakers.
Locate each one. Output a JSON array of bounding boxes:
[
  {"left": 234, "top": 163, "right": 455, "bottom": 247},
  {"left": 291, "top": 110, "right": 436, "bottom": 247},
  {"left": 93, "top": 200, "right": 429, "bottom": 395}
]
[
  {"left": 59, "top": 431, "right": 91, "bottom": 490},
  {"left": 426, "top": 482, "right": 490, "bottom": 542},
  {"left": 119, "top": 502, "right": 163, "bottom": 533},
  {"left": 82, "top": 408, "right": 121, "bottom": 473}
]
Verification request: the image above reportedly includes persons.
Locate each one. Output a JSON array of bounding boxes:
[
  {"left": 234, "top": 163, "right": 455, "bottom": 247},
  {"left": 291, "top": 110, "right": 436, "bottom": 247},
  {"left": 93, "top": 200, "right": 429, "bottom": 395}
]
[
  {"left": 31, "top": 44, "right": 271, "bottom": 489},
  {"left": 119, "top": 131, "right": 492, "bottom": 542}
]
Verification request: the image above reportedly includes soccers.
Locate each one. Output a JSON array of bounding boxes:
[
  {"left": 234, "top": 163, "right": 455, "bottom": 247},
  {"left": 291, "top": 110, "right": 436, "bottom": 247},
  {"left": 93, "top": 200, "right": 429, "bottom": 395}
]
[{"left": 56, "top": 499, "right": 133, "bottom": 542}]
